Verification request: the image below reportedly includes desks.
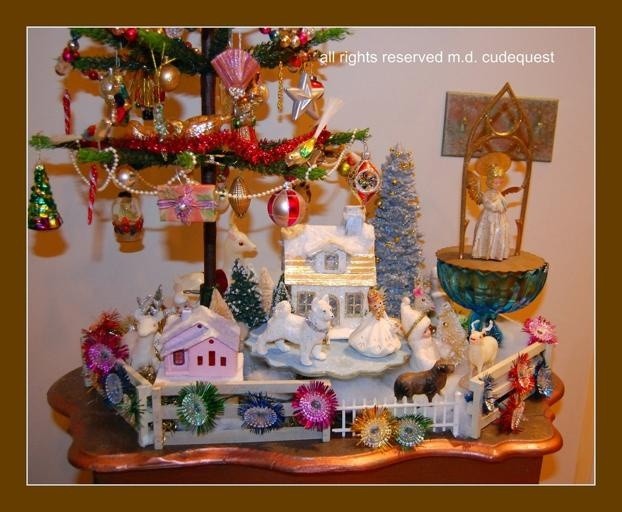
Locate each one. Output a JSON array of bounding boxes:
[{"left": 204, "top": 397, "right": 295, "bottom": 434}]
[{"left": 43, "top": 358, "right": 569, "bottom": 485}]
